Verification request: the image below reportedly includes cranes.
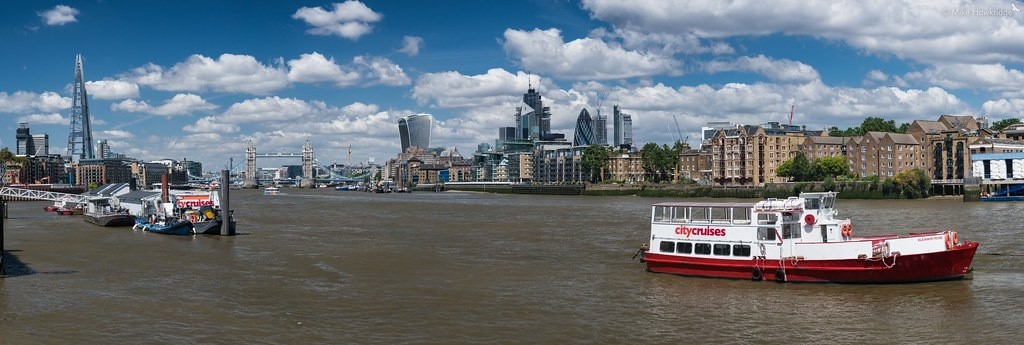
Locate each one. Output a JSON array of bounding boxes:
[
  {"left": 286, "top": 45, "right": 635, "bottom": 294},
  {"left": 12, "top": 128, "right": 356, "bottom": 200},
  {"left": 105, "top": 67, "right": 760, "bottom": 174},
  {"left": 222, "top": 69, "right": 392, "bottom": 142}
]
[
  {"left": 788, "top": 104, "right": 795, "bottom": 126},
  {"left": 672, "top": 115, "right": 689, "bottom": 152}
]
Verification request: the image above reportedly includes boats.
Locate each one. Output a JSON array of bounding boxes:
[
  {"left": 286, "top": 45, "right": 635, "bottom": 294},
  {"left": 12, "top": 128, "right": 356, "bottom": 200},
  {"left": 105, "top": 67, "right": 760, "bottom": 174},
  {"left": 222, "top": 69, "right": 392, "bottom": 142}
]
[
  {"left": 263, "top": 186, "right": 280, "bottom": 196},
  {"left": 335, "top": 181, "right": 412, "bottom": 194},
  {"left": 979, "top": 177, "right": 1024, "bottom": 202},
  {"left": 42, "top": 180, "right": 237, "bottom": 237},
  {"left": 631, "top": 190, "right": 982, "bottom": 284}
]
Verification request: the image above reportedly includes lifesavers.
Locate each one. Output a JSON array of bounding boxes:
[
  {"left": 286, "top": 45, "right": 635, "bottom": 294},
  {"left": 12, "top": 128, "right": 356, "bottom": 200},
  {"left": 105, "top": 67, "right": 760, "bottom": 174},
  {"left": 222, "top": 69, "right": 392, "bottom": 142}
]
[
  {"left": 953, "top": 231, "right": 958, "bottom": 245},
  {"left": 841, "top": 224, "right": 848, "bottom": 237},
  {"left": 775, "top": 270, "right": 785, "bottom": 281},
  {"left": 847, "top": 223, "right": 852, "bottom": 236},
  {"left": 752, "top": 269, "right": 762, "bottom": 280},
  {"left": 945, "top": 233, "right": 952, "bottom": 249}
]
